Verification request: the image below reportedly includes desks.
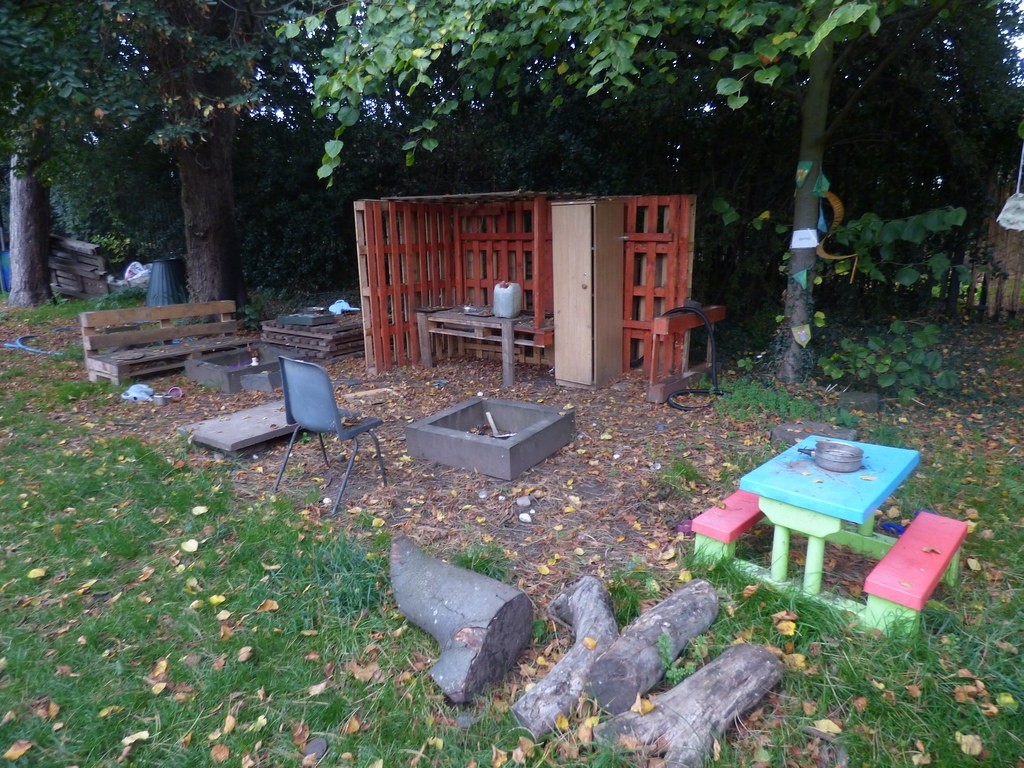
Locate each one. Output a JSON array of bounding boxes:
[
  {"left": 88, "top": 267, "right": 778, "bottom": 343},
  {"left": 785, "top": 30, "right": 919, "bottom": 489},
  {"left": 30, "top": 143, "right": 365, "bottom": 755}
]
[
  {"left": 415, "top": 306, "right": 554, "bottom": 388},
  {"left": 737, "top": 435, "right": 920, "bottom": 618}
]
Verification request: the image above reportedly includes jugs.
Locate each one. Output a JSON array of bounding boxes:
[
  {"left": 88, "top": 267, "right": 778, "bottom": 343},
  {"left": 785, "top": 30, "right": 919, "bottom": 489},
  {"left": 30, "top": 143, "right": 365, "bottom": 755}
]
[
  {"left": 121, "top": 384, "right": 155, "bottom": 402},
  {"left": 328, "top": 300, "right": 360, "bottom": 316}
]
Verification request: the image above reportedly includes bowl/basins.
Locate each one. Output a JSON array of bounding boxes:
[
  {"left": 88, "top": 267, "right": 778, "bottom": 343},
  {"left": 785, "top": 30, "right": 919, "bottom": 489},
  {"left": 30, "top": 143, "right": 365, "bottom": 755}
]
[
  {"left": 168, "top": 387, "right": 184, "bottom": 398},
  {"left": 302, "top": 306, "right": 327, "bottom": 315}
]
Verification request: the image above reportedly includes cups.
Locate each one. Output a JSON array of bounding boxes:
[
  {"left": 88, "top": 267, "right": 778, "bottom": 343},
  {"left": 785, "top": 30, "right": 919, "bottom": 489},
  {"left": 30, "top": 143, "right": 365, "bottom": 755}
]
[{"left": 153, "top": 393, "right": 172, "bottom": 405}]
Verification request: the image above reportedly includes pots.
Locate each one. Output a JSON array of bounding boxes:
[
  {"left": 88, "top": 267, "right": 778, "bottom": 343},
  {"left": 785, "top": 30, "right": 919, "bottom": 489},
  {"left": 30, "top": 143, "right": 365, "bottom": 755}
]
[{"left": 797, "top": 441, "right": 864, "bottom": 472}]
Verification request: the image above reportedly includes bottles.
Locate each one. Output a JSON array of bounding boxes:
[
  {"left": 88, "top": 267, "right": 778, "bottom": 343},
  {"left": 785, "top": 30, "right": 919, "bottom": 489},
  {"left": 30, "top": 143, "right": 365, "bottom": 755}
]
[{"left": 251, "top": 358, "right": 259, "bottom": 366}]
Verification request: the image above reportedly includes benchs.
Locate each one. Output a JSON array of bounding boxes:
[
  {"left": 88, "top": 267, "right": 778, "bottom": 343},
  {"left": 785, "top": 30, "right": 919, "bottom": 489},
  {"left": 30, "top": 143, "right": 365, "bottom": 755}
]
[
  {"left": 864, "top": 512, "right": 968, "bottom": 640},
  {"left": 80, "top": 298, "right": 253, "bottom": 385},
  {"left": 692, "top": 490, "right": 767, "bottom": 565}
]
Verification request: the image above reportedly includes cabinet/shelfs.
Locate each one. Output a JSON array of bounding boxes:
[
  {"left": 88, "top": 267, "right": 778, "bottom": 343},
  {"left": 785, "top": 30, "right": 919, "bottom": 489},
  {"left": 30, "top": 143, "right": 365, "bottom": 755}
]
[{"left": 551, "top": 199, "right": 625, "bottom": 392}]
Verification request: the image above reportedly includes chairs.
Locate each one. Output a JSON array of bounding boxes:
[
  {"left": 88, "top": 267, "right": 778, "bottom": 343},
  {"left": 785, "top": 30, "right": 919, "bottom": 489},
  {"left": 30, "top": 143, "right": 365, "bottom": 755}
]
[{"left": 273, "top": 356, "right": 387, "bottom": 513}]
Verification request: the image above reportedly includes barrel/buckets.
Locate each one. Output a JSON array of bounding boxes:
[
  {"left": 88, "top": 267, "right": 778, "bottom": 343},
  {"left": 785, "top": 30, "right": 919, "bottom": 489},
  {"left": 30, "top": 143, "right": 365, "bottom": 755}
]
[
  {"left": 493, "top": 281, "right": 521, "bottom": 318},
  {"left": 1, "top": 250, "right": 11, "bottom": 291}
]
